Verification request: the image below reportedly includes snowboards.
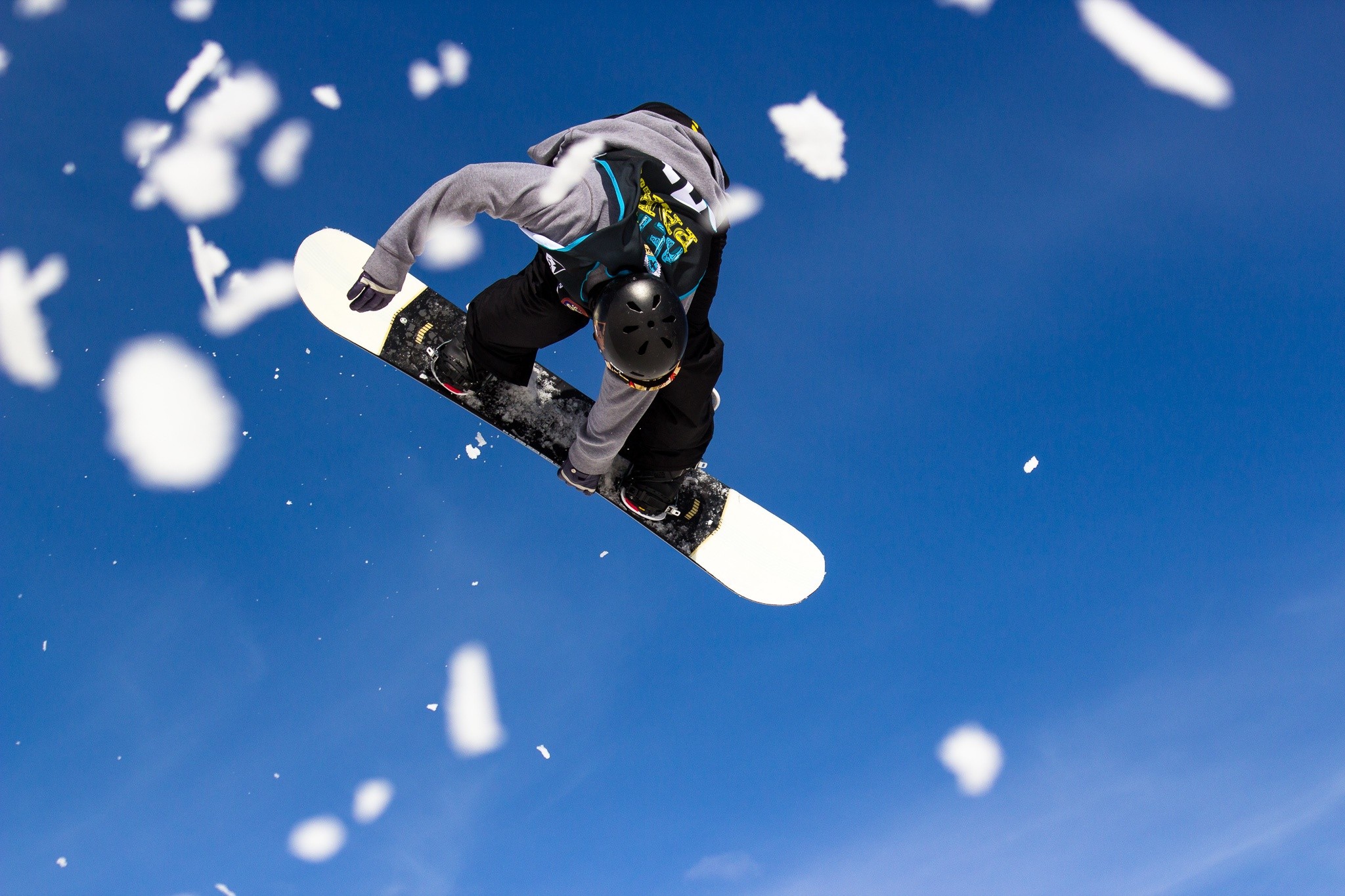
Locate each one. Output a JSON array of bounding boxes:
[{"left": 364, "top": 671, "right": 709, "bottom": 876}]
[{"left": 291, "top": 226, "right": 827, "bottom": 607}]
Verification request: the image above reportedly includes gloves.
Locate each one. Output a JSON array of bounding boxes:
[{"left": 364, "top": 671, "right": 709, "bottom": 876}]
[
  {"left": 557, "top": 459, "right": 601, "bottom": 495},
  {"left": 346, "top": 270, "right": 397, "bottom": 313}
]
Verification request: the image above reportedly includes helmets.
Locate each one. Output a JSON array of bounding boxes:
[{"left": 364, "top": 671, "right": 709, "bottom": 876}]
[{"left": 593, "top": 272, "right": 688, "bottom": 382}]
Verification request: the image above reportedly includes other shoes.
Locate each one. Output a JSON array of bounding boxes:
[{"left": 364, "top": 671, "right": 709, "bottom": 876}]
[
  {"left": 438, "top": 327, "right": 477, "bottom": 383},
  {"left": 626, "top": 463, "right": 686, "bottom": 516}
]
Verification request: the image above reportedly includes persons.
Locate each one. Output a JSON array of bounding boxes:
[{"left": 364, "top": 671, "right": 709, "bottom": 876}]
[{"left": 346, "top": 103, "right": 729, "bottom": 520}]
